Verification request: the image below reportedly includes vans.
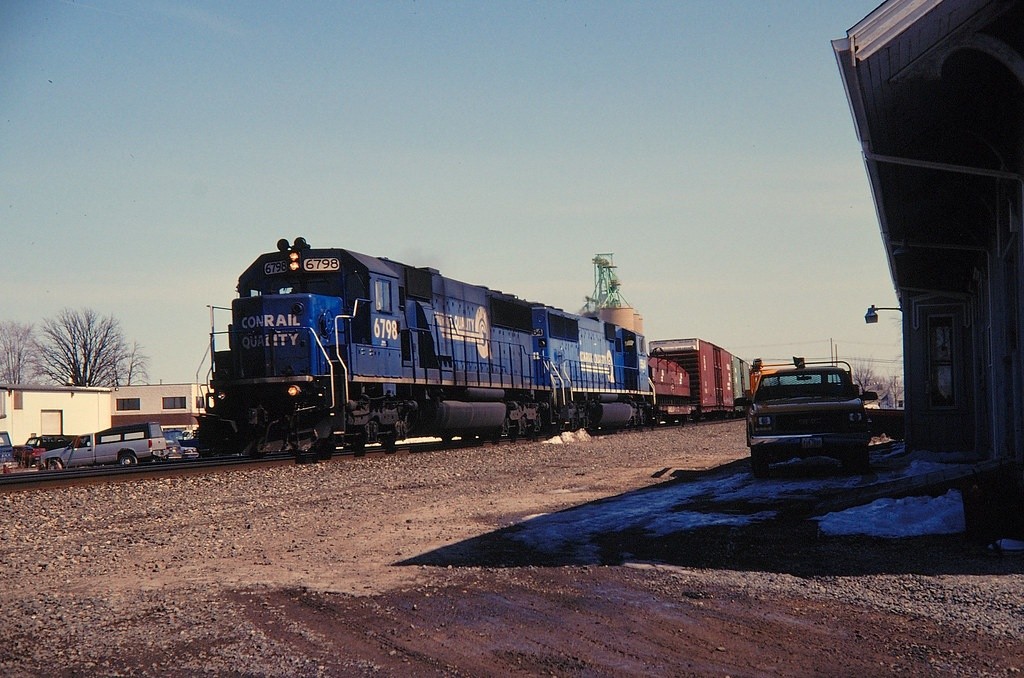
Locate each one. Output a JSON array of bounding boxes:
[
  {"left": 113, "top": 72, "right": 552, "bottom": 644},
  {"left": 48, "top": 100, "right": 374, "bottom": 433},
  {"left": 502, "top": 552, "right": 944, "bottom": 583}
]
[{"left": 0, "top": 431, "right": 14, "bottom": 463}]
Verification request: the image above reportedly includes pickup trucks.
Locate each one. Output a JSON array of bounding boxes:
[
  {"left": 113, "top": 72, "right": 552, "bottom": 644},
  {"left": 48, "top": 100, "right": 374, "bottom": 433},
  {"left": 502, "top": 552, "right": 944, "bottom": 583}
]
[
  {"left": 12, "top": 433, "right": 73, "bottom": 467},
  {"left": 36, "top": 420, "right": 168, "bottom": 471},
  {"left": 744, "top": 355, "right": 878, "bottom": 476}
]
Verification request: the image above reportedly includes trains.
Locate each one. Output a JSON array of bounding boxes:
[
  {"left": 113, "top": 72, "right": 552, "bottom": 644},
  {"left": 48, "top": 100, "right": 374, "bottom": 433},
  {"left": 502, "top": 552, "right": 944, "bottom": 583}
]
[{"left": 186, "top": 235, "right": 774, "bottom": 464}]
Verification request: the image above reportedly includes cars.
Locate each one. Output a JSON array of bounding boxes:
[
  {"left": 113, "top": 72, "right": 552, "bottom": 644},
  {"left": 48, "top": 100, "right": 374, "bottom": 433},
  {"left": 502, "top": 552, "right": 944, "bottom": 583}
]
[{"left": 149, "top": 429, "right": 199, "bottom": 459}]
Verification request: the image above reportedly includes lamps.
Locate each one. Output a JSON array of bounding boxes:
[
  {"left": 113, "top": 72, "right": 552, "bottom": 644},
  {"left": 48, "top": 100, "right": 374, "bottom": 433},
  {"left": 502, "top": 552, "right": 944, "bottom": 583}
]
[{"left": 865, "top": 306, "right": 902, "bottom": 324}]
